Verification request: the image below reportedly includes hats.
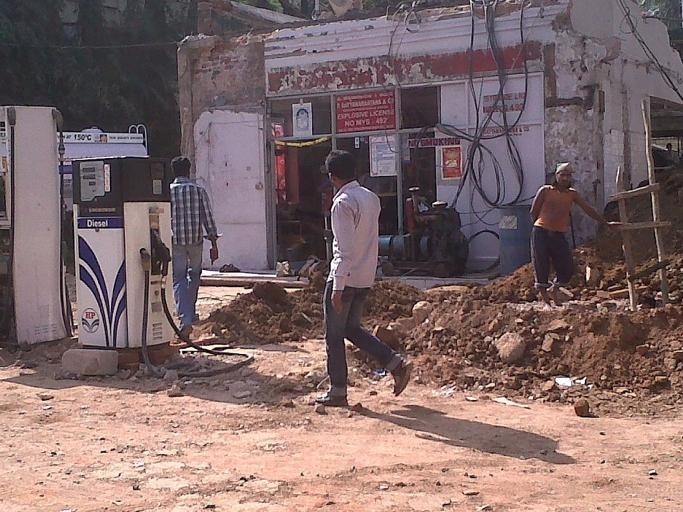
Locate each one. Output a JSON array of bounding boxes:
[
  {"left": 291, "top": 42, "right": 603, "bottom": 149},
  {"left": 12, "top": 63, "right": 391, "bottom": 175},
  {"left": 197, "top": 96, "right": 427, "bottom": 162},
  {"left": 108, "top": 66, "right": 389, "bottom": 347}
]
[{"left": 556, "top": 162, "right": 572, "bottom": 174}]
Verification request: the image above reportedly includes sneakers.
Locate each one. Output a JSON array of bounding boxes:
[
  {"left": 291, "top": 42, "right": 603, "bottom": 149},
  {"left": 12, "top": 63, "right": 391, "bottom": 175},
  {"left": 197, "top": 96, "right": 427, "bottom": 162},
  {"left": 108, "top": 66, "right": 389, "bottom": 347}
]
[
  {"left": 316, "top": 395, "right": 349, "bottom": 407},
  {"left": 175, "top": 325, "right": 193, "bottom": 340},
  {"left": 391, "top": 360, "right": 412, "bottom": 396},
  {"left": 191, "top": 313, "right": 200, "bottom": 324}
]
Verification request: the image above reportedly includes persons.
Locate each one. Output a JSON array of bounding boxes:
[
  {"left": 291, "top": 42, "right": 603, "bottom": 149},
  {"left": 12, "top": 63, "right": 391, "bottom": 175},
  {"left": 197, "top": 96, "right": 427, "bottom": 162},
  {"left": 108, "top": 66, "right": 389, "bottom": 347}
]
[
  {"left": 529, "top": 163, "right": 608, "bottom": 302},
  {"left": 168, "top": 155, "right": 218, "bottom": 339},
  {"left": 657, "top": 142, "right": 680, "bottom": 167},
  {"left": 314, "top": 150, "right": 412, "bottom": 407}
]
